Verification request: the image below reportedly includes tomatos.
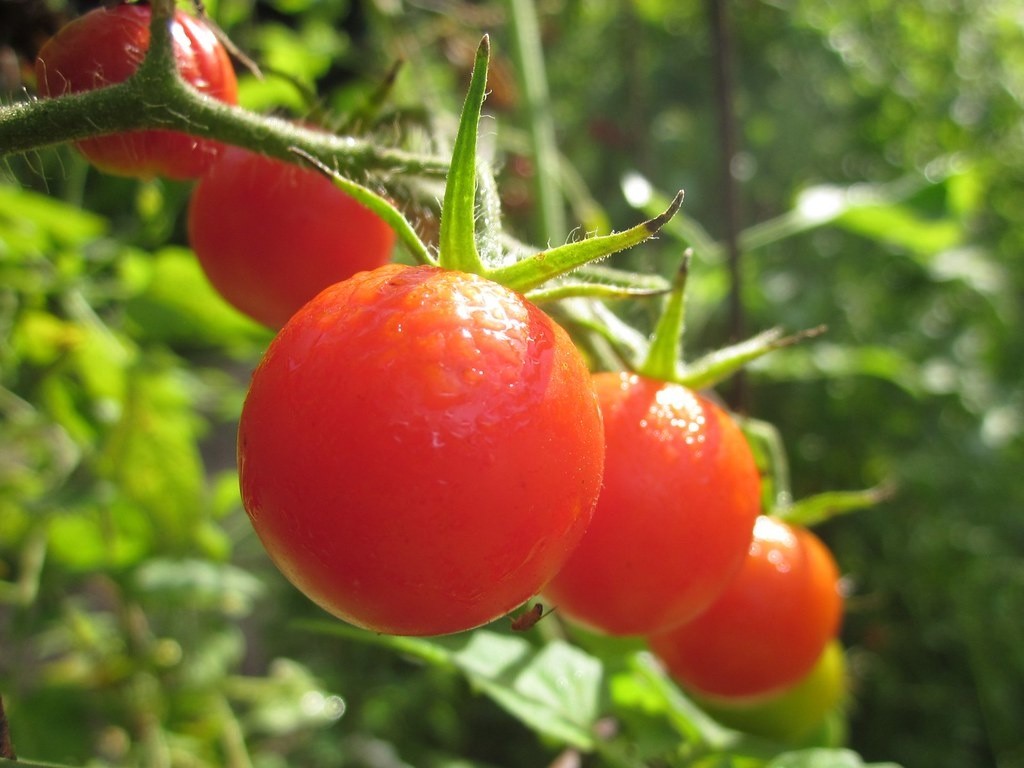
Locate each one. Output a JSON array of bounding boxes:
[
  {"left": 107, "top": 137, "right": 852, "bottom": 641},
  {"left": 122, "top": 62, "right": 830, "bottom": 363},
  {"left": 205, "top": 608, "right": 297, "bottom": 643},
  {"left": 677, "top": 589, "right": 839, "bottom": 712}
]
[{"left": 34, "top": 4, "right": 896, "bottom": 700}]
